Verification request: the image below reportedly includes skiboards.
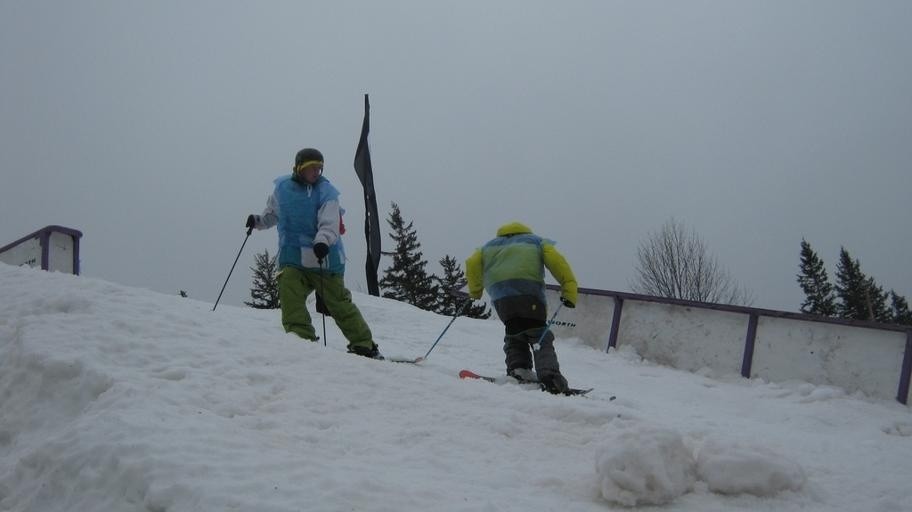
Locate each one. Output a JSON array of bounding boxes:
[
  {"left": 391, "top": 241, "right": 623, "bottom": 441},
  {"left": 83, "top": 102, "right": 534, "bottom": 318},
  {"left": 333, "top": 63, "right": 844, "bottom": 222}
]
[
  {"left": 379, "top": 358, "right": 424, "bottom": 363},
  {"left": 459, "top": 368, "right": 594, "bottom": 396}
]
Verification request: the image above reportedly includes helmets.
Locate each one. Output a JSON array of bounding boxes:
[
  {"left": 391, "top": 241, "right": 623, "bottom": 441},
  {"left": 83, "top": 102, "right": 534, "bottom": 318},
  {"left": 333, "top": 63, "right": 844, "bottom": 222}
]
[{"left": 294, "top": 148, "right": 325, "bottom": 172}]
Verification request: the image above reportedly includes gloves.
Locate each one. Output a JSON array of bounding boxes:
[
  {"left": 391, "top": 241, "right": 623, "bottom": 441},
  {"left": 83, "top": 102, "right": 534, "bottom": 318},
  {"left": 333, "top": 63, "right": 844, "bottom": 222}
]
[
  {"left": 245, "top": 213, "right": 256, "bottom": 229},
  {"left": 313, "top": 242, "right": 329, "bottom": 259}
]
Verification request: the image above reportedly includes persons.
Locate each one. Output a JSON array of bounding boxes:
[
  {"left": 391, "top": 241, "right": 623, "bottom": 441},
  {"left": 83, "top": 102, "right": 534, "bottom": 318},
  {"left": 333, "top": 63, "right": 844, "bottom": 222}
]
[
  {"left": 246, "top": 148, "right": 384, "bottom": 360},
  {"left": 464, "top": 222, "right": 578, "bottom": 395}
]
